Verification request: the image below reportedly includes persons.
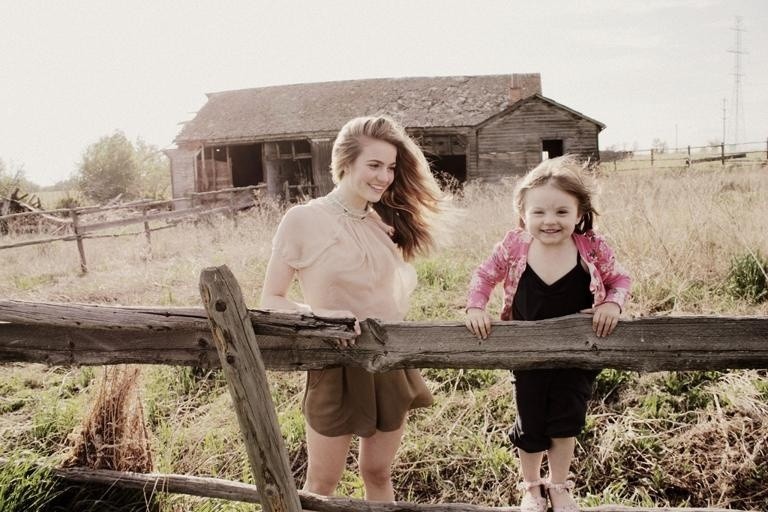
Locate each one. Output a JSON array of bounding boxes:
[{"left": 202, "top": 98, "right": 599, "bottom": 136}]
[
  {"left": 465, "top": 159, "right": 632, "bottom": 512},
  {"left": 261, "top": 116, "right": 448, "bottom": 502}
]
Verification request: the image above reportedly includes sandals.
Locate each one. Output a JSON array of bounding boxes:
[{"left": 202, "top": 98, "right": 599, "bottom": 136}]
[
  {"left": 517, "top": 480, "right": 548, "bottom": 512},
  {"left": 545, "top": 474, "right": 578, "bottom": 512}
]
